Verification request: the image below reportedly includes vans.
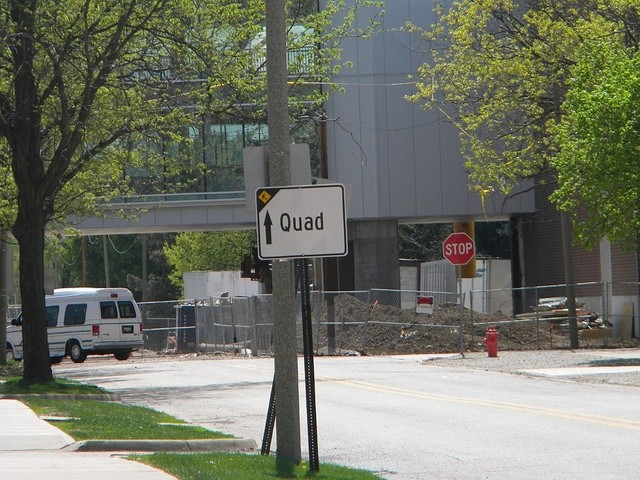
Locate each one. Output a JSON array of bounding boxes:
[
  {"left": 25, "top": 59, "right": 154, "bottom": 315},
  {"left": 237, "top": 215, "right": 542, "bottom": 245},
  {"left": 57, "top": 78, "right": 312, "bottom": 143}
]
[{"left": 6, "top": 287, "right": 143, "bottom": 363}]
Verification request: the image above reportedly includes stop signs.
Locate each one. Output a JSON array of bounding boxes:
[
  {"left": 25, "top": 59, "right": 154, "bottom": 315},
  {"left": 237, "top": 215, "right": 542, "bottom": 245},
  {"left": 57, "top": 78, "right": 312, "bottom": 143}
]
[{"left": 442, "top": 232, "right": 475, "bottom": 266}]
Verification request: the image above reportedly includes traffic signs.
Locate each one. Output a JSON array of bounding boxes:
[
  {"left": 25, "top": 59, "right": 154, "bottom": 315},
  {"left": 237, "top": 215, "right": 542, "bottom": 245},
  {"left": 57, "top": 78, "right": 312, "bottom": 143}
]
[{"left": 255, "top": 183, "right": 348, "bottom": 261}]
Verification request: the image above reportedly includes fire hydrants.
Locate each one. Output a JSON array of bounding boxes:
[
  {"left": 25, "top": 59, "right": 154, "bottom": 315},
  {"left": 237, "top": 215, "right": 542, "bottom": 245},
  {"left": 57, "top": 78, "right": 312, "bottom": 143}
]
[{"left": 482, "top": 325, "right": 501, "bottom": 357}]
